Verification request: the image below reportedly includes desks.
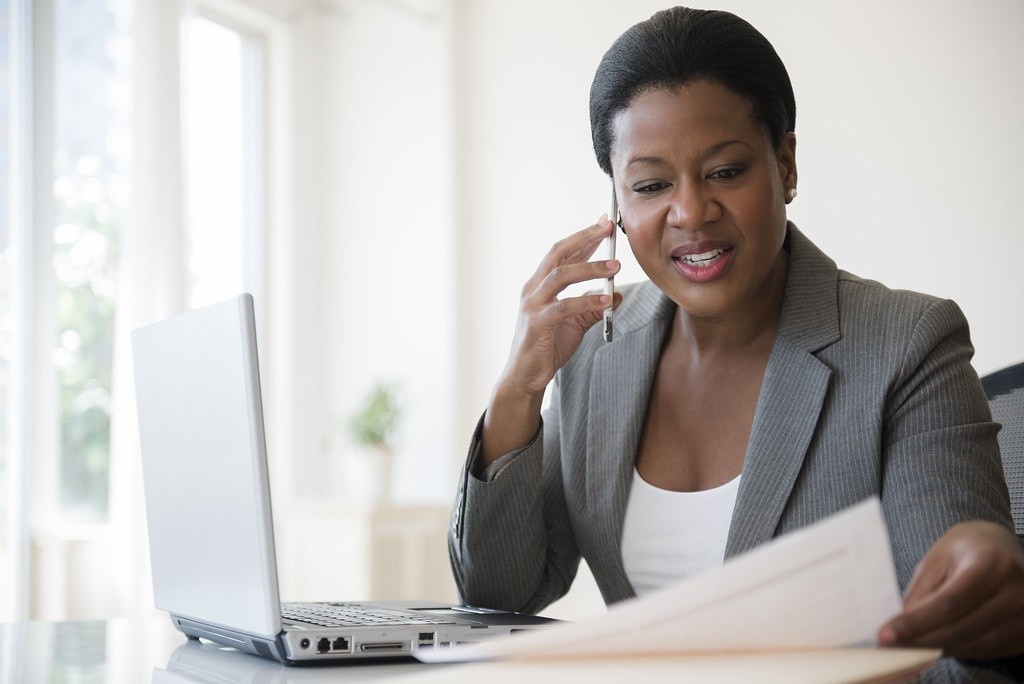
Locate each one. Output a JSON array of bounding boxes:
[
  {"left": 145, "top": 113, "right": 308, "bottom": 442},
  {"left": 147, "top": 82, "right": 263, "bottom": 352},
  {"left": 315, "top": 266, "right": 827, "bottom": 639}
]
[{"left": 0, "top": 618, "right": 447, "bottom": 684}]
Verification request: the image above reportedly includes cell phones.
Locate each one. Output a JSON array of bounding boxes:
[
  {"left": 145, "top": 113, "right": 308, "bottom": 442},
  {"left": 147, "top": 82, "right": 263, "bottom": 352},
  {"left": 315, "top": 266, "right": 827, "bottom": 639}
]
[{"left": 603, "top": 179, "right": 618, "bottom": 343}]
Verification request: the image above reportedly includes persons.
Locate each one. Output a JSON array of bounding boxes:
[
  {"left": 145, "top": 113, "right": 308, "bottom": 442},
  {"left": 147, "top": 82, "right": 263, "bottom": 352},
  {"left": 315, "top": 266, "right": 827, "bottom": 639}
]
[{"left": 448, "top": 7, "right": 1024, "bottom": 684}]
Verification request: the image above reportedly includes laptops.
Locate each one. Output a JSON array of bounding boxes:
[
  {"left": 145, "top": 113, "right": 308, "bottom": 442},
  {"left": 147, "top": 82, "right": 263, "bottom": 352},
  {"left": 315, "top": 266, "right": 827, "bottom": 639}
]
[{"left": 131, "top": 292, "right": 561, "bottom": 662}]
